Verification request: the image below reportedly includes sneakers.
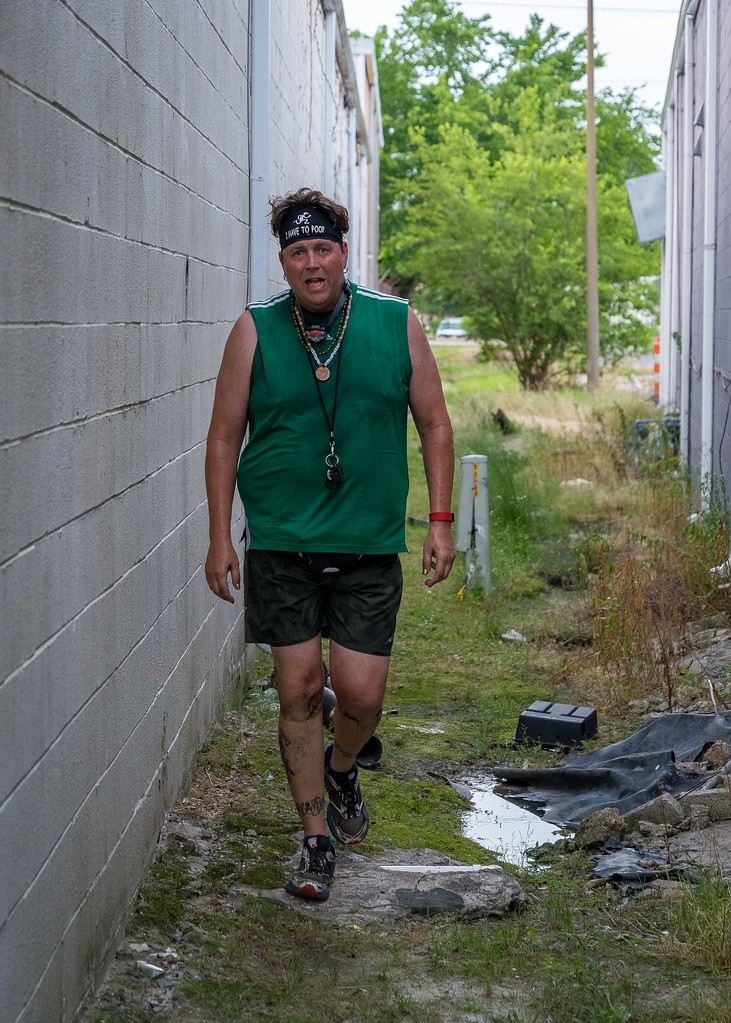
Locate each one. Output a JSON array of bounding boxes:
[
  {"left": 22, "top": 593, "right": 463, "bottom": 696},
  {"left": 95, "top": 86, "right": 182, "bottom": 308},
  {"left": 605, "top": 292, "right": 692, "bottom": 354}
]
[
  {"left": 284, "top": 838, "right": 337, "bottom": 902},
  {"left": 323, "top": 743, "right": 370, "bottom": 847}
]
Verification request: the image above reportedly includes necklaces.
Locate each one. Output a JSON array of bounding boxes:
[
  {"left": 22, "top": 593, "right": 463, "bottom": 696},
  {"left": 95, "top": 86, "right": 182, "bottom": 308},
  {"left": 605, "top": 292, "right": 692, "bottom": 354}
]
[{"left": 289, "top": 284, "right": 353, "bottom": 494}]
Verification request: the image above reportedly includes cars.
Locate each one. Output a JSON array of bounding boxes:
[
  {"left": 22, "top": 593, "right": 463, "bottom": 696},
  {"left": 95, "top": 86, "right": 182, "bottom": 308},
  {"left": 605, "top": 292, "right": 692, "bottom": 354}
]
[{"left": 436, "top": 316, "right": 468, "bottom": 338}]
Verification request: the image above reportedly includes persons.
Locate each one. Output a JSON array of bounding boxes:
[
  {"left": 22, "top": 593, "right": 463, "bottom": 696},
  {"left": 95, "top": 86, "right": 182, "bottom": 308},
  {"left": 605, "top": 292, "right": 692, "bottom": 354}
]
[{"left": 205, "top": 190, "right": 456, "bottom": 900}]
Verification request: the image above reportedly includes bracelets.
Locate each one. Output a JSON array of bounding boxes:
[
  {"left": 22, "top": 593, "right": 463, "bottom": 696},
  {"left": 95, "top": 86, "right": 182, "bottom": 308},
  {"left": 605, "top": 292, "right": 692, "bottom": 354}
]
[{"left": 428, "top": 512, "right": 455, "bottom": 523}]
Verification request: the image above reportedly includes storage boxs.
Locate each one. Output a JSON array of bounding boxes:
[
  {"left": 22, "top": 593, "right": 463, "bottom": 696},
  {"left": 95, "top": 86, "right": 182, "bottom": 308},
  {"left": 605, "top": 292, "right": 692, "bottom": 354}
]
[{"left": 513, "top": 701, "right": 598, "bottom": 750}]
